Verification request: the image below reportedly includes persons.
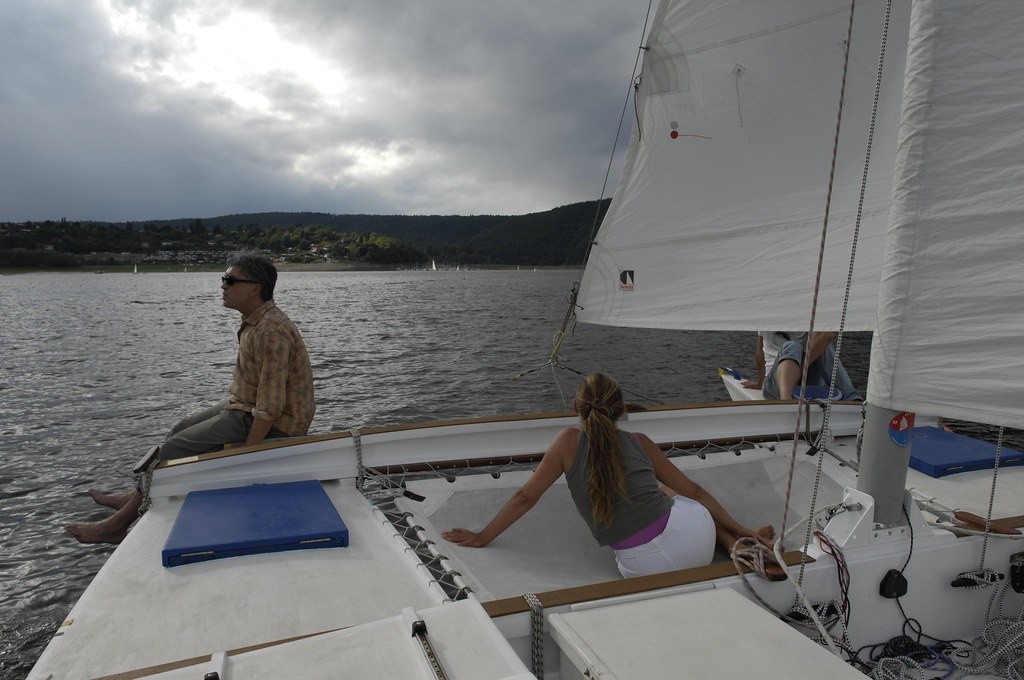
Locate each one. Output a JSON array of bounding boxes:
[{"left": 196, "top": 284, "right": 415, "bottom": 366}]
[
  {"left": 62, "top": 254, "right": 317, "bottom": 546},
  {"left": 740, "top": 331, "right": 865, "bottom": 403},
  {"left": 440, "top": 372, "right": 777, "bottom": 581}
]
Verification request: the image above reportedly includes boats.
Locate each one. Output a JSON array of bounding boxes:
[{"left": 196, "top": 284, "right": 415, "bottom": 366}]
[{"left": 716, "top": 364, "right": 847, "bottom": 402}]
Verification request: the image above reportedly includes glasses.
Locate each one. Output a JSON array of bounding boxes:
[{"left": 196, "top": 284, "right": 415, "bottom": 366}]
[{"left": 222, "top": 276, "right": 262, "bottom": 286}]
